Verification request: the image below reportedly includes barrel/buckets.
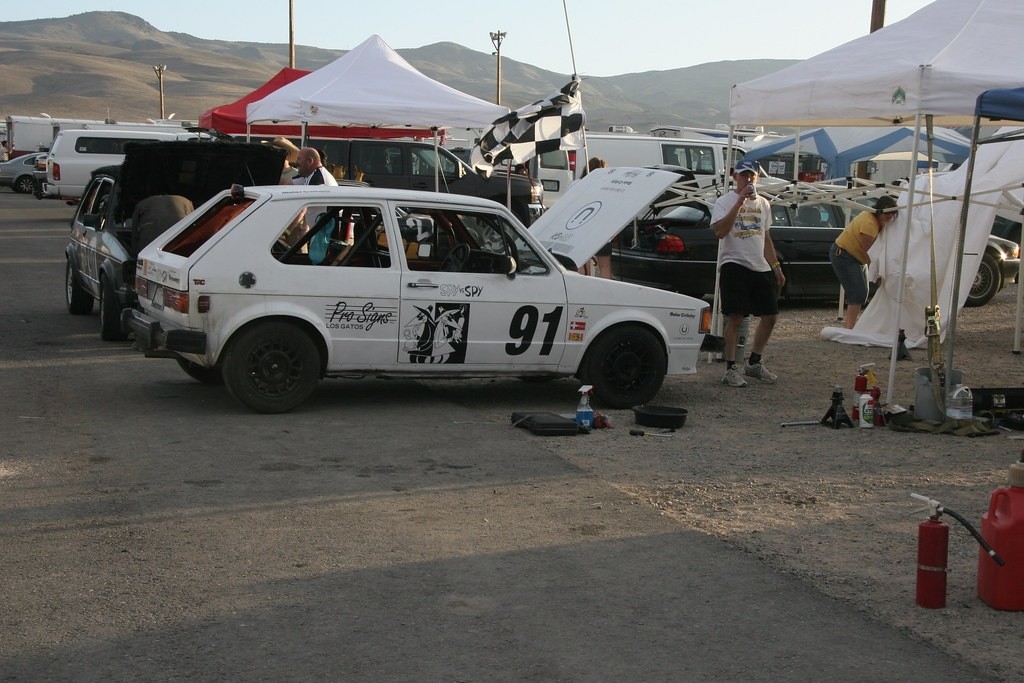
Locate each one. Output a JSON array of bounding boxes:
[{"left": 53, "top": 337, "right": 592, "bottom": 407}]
[
  {"left": 913, "top": 366, "right": 964, "bottom": 423},
  {"left": 977, "top": 449, "right": 1024, "bottom": 611}
]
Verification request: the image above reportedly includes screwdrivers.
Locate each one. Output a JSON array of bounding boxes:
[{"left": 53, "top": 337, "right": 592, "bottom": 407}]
[{"left": 629, "top": 430, "right": 674, "bottom": 437}]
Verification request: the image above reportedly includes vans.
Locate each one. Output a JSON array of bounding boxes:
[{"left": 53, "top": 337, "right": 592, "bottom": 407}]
[{"left": 539, "top": 132, "right": 789, "bottom": 213}]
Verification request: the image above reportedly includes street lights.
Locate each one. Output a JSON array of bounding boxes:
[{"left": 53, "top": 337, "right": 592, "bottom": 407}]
[
  {"left": 153, "top": 65, "right": 167, "bottom": 119},
  {"left": 490, "top": 32, "right": 507, "bottom": 104}
]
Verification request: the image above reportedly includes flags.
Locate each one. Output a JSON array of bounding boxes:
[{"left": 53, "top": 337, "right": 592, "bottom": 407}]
[{"left": 470, "top": 74, "right": 586, "bottom": 183}]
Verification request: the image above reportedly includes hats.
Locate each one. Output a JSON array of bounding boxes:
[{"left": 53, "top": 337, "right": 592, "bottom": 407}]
[
  {"left": 260, "top": 137, "right": 300, "bottom": 162},
  {"left": 735, "top": 158, "right": 760, "bottom": 176}
]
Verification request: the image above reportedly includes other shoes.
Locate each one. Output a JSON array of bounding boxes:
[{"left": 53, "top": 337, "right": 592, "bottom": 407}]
[{"left": 132, "top": 342, "right": 148, "bottom": 351}]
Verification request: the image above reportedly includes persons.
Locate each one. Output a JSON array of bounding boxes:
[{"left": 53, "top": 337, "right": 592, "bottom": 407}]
[
  {"left": 273, "top": 137, "right": 343, "bottom": 253},
  {"left": 0, "top": 141, "right": 15, "bottom": 162},
  {"left": 710, "top": 158, "right": 786, "bottom": 387},
  {"left": 132, "top": 195, "right": 194, "bottom": 351},
  {"left": 829, "top": 196, "right": 898, "bottom": 329},
  {"left": 565, "top": 157, "right": 613, "bottom": 279}
]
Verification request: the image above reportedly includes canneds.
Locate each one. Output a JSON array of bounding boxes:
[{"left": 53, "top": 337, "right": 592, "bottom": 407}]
[{"left": 746, "top": 184, "right": 757, "bottom": 200}]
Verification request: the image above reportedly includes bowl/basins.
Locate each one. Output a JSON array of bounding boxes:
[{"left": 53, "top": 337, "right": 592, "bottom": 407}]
[
  {"left": 859, "top": 392, "right": 873, "bottom": 428},
  {"left": 631, "top": 404, "right": 689, "bottom": 427}
]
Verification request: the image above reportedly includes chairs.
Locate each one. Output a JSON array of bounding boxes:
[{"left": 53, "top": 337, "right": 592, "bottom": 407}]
[
  {"left": 800, "top": 207, "right": 831, "bottom": 228},
  {"left": 348, "top": 221, "right": 381, "bottom": 267},
  {"left": 310, "top": 213, "right": 345, "bottom": 266}
]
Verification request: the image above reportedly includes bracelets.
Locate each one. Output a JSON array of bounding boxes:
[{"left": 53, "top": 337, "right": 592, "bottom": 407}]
[{"left": 772, "top": 261, "right": 780, "bottom": 269}]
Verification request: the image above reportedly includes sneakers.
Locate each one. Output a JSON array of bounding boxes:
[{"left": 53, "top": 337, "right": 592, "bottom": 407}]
[
  {"left": 722, "top": 366, "right": 747, "bottom": 387},
  {"left": 743, "top": 359, "right": 777, "bottom": 384}
]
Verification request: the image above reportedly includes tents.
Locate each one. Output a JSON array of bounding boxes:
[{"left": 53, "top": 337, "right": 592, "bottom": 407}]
[
  {"left": 199, "top": 34, "right": 511, "bottom": 211},
  {"left": 729, "top": 0, "right": 1024, "bottom": 405}
]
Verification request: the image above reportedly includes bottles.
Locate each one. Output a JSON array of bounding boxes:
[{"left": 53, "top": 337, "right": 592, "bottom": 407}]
[
  {"left": 747, "top": 183, "right": 756, "bottom": 197},
  {"left": 946, "top": 384, "right": 973, "bottom": 419}
]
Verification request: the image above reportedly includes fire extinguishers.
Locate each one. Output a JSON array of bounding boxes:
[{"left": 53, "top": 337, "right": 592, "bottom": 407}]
[
  {"left": 910, "top": 492, "right": 1007, "bottom": 608},
  {"left": 851, "top": 362, "right": 875, "bottom": 420}
]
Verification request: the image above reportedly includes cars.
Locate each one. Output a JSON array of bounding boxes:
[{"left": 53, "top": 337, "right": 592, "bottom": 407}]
[
  {"left": 612, "top": 191, "right": 1020, "bottom": 308},
  {"left": 64, "top": 142, "right": 289, "bottom": 342},
  {"left": 121, "top": 165, "right": 711, "bottom": 415},
  {"left": 0, "top": 153, "right": 48, "bottom": 195}
]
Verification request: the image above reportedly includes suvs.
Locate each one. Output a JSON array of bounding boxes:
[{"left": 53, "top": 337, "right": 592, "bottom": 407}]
[
  {"left": 41, "top": 127, "right": 186, "bottom": 205},
  {"left": 282, "top": 137, "right": 549, "bottom": 254}
]
